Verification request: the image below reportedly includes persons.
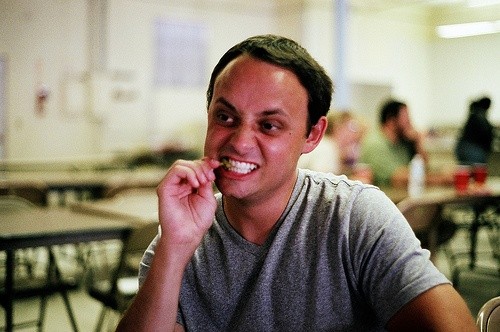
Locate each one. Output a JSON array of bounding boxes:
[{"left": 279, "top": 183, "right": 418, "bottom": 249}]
[
  {"left": 359, "top": 98, "right": 452, "bottom": 188},
  {"left": 455, "top": 98, "right": 496, "bottom": 165},
  {"left": 297, "top": 111, "right": 374, "bottom": 184},
  {"left": 114, "top": 34, "right": 480, "bottom": 332}
]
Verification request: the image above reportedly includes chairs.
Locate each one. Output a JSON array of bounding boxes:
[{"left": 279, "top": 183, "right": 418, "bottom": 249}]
[
  {"left": 0, "top": 195, "right": 79, "bottom": 332},
  {"left": 86, "top": 222, "right": 160, "bottom": 332},
  {"left": 396, "top": 191, "right": 457, "bottom": 261}
]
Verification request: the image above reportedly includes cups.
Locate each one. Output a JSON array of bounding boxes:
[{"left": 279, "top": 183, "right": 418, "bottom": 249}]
[
  {"left": 452, "top": 168, "right": 471, "bottom": 190},
  {"left": 472, "top": 163, "right": 489, "bottom": 185}
]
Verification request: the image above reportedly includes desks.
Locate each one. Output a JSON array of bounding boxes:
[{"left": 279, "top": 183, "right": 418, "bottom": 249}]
[{"left": 0, "top": 162, "right": 500, "bottom": 332}]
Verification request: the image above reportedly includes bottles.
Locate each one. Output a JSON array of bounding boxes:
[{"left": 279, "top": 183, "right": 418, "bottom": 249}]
[{"left": 408, "top": 155, "right": 428, "bottom": 198}]
